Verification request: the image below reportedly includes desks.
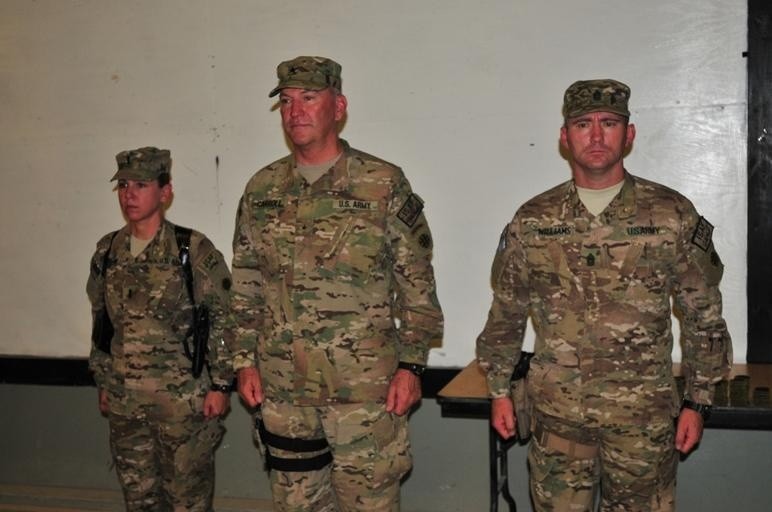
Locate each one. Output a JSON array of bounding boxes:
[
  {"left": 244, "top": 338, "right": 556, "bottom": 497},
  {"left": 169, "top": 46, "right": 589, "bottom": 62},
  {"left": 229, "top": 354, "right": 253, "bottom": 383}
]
[{"left": 437, "top": 358, "right": 772, "bottom": 512}]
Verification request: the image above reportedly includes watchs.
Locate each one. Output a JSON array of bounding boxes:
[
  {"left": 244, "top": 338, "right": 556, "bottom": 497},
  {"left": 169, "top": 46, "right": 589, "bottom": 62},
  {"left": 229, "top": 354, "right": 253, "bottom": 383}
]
[
  {"left": 682, "top": 399, "right": 714, "bottom": 420},
  {"left": 211, "top": 384, "right": 231, "bottom": 393},
  {"left": 398, "top": 362, "right": 426, "bottom": 377}
]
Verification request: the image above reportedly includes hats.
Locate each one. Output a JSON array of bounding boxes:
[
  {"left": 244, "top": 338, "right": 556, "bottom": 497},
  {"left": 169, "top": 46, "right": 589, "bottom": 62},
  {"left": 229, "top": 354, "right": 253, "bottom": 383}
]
[
  {"left": 108, "top": 146, "right": 173, "bottom": 186},
  {"left": 268, "top": 55, "right": 343, "bottom": 105},
  {"left": 562, "top": 78, "right": 632, "bottom": 121}
]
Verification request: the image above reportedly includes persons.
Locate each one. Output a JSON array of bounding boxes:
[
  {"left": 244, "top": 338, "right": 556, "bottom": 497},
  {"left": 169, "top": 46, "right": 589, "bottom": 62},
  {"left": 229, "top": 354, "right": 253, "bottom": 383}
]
[
  {"left": 86, "top": 148, "right": 231, "bottom": 510},
  {"left": 477, "top": 78, "right": 733, "bottom": 512},
  {"left": 228, "top": 57, "right": 442, "bottom": 512}
]
